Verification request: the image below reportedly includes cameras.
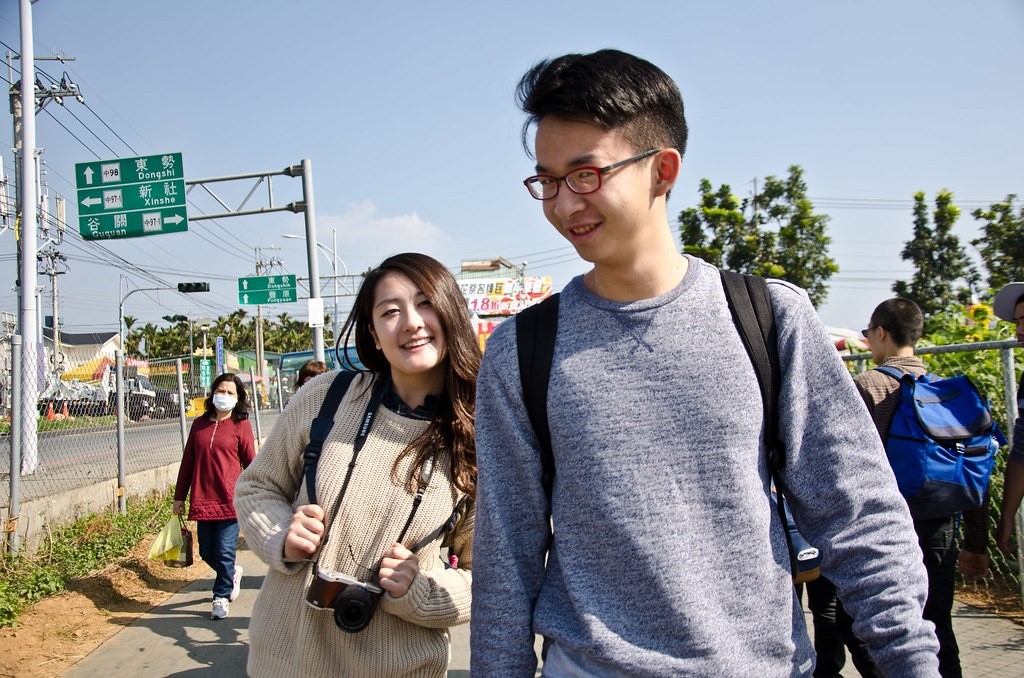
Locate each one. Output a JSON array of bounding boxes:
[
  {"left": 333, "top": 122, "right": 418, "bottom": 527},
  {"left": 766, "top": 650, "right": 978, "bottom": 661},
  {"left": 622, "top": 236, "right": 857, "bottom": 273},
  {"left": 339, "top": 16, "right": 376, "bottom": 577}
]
[{"left": 304, "top": 570, "right": 385, "bottom": 633}]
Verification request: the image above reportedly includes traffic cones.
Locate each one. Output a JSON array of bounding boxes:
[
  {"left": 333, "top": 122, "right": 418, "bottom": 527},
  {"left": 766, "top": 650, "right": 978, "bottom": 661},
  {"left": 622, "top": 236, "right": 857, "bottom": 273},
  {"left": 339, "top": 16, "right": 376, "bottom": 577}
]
[
  {"left": 47, "top": 402, "right": 56, "bottom": 422},
  {"left": 63, "top": 401, "right": 68, "bottom": 420}
]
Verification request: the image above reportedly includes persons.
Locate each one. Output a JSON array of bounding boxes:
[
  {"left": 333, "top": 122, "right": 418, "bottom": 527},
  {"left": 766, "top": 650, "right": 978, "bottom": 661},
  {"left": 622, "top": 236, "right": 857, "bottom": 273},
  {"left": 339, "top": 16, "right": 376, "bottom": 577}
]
[
  {"left": 233, "top": 252, "right": 483, "bottom": 678},
  {"left": 993, "top": 282, "right": 1024, "bottom": 557},
  {"left": 794, "top": 298, "right": 990, "bottom": 678},
  {"left": 470, "top": 48, "right": 944, "bottom": 678},
  {"left": 173, "top": 373, "right": 256, "bottom": 620},
  {"left": 256, "top": 381, "right": 264, "bottom": 410},
  {"left": 296, "top": 361, "right": 327, "bottom": 391}
]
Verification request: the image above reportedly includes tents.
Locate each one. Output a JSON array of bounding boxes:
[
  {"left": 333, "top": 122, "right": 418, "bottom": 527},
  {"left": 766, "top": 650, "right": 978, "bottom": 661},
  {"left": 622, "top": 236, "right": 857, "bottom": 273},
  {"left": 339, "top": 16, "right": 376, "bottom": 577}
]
[{"left": 61, "top": 356, "right": 115, "bottom": 381}]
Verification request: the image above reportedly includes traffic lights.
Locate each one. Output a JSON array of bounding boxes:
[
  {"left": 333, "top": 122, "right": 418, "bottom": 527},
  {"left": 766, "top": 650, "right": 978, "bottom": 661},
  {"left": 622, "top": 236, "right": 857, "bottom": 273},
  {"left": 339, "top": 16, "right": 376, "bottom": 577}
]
[{"left": 177, "top": 282, "right": 209, "bottom": 293}]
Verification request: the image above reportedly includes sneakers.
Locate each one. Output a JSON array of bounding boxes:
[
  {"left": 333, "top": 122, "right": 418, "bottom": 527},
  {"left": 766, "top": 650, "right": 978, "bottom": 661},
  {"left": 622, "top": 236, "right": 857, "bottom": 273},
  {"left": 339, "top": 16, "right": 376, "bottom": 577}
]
[
  {"left": 227, "top": 565, "right": 244, "bottom": 603},
  {"left": 211, "top": 593, "right": 230, "bottom": 619}
]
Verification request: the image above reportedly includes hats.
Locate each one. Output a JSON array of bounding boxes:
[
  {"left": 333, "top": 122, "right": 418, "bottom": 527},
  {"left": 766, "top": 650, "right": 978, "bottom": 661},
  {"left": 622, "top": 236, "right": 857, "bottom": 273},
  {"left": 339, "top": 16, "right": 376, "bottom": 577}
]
[{"left": 993, "top": 281, "right": 1024, "bottom": 322}]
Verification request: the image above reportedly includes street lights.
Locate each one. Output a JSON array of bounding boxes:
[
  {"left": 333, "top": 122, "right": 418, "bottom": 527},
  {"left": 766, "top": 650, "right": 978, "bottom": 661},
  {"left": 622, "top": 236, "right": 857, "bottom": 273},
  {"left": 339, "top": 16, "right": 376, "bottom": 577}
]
[{"left": 281, "top": 227, "right": 348, "bottom": 370}]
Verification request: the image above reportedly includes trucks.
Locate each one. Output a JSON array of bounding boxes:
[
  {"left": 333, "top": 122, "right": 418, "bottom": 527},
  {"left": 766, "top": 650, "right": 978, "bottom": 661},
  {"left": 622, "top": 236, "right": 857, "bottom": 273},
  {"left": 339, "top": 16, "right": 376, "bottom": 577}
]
[{"left": 39, "top": 373, "right": 192, "bottom": 419}]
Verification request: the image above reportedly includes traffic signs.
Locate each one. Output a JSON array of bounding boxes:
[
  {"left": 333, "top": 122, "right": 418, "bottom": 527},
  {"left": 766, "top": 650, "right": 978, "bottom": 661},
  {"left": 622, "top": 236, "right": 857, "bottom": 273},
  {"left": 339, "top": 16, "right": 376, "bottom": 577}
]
[
  {"left": 237, "top": 273, "right": 297, "bottom": 306},
  {"left": 74, "top": 152, "right": 189, "bottom": 242}
]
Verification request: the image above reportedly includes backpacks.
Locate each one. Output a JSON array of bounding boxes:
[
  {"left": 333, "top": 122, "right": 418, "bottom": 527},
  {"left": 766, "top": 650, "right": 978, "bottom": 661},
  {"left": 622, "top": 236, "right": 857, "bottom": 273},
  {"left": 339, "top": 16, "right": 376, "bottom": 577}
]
[{"left": 874, "top": 365, "right": 1010, "bottom": 522}]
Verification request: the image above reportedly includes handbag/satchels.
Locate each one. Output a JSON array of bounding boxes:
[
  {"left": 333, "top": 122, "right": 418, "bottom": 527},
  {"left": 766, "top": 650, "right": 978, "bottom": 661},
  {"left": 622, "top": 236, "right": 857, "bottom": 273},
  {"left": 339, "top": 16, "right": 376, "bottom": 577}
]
[{"left": 148, "top": 512, "right": 194, "bottom": 568}]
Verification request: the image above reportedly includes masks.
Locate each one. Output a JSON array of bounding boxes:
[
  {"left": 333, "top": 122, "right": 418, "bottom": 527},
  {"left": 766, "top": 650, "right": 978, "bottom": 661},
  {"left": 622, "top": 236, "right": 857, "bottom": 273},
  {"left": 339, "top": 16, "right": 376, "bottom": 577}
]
[{"left": 212, "top": 393, "right": 240, "bottom": 411}]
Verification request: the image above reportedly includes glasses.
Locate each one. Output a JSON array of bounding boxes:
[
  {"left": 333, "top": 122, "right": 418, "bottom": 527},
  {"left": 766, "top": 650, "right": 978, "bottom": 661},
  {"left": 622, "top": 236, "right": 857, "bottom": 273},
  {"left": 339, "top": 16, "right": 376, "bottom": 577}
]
[
  {"left": 524, "top": 145, "right": 668, "bottom": 200},
  {"left": 861, "top": 324, "right": 890, "bottom": 338}
]
